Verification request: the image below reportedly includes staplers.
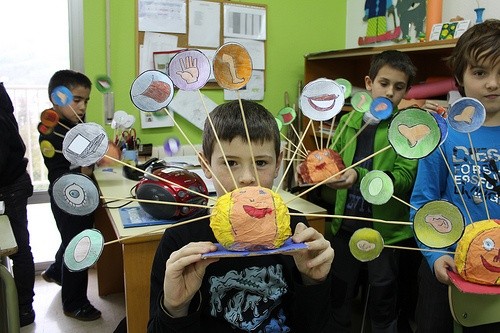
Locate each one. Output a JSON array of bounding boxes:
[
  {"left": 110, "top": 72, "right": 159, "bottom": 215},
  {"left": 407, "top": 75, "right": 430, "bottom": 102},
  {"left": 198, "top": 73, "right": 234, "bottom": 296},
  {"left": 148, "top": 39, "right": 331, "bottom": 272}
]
[{"left": 139, "top": 157, "right": 165, "bottom": 171}]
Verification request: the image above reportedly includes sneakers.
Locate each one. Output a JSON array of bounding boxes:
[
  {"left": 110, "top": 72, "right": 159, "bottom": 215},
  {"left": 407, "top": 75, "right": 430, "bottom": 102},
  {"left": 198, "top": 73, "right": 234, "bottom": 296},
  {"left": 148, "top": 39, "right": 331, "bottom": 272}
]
[
  {"left": 40, "top": 270, "right": 63, "bottom": 287},
  {"left": 63, "top": 303, "right": 102, "bottom": 321}
]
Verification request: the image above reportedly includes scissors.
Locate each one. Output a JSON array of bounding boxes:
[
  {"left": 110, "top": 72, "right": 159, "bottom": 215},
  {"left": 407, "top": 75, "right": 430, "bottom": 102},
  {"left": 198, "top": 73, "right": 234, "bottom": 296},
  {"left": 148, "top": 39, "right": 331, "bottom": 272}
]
[{"left": 121, "top": 126, "right": 137, "bottom": 141}]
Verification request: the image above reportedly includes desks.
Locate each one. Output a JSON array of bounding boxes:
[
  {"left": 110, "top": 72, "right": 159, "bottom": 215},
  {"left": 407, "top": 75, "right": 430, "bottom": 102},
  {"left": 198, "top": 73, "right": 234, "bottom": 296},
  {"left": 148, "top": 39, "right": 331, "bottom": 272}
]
[
  {"left": 93, "top": 156, "right": 327, "bottom": 333},
  {"left": 0, "top": 215, "right": 21, "bottom": 333}
]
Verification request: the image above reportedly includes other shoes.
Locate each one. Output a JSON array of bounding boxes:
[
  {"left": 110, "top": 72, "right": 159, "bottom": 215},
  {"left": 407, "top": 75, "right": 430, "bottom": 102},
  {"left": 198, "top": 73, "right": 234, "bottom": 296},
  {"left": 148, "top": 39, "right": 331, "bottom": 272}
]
[{"left": 20, "top": 309, "right": 35, "bottom": 327}]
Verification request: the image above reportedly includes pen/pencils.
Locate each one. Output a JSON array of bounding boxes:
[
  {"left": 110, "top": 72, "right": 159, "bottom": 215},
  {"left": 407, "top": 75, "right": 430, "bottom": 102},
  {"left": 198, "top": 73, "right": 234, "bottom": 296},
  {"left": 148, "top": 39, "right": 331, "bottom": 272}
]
[{"left": 121, "top": 135, "right": 136, "bottom": 150}]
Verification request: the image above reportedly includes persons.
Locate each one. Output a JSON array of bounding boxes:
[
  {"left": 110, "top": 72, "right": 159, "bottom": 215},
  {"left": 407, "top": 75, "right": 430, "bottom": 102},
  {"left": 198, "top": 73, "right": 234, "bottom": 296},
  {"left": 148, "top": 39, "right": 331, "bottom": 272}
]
[
  {"left": 294, "top": 51, "right": 418, "bottom": 333},
  {"left": 0, "top": 81, "right": 36, "bottom": 328},
  {"left": 39, "top": 70, "right": 104, "bottom": 321},
  {"left": 410, "top": 20, "right": 500, "bottom": 333},
  {"left": 146, "top": 98, "right": 341, "bottom": 333}
]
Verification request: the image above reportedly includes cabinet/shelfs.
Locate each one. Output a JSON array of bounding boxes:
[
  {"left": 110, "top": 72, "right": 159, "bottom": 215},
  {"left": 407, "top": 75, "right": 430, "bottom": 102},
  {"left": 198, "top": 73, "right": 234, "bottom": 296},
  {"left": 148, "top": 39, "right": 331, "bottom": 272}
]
[{"left": 298, "top": 37, "right": 459, "bottom": 198}]
